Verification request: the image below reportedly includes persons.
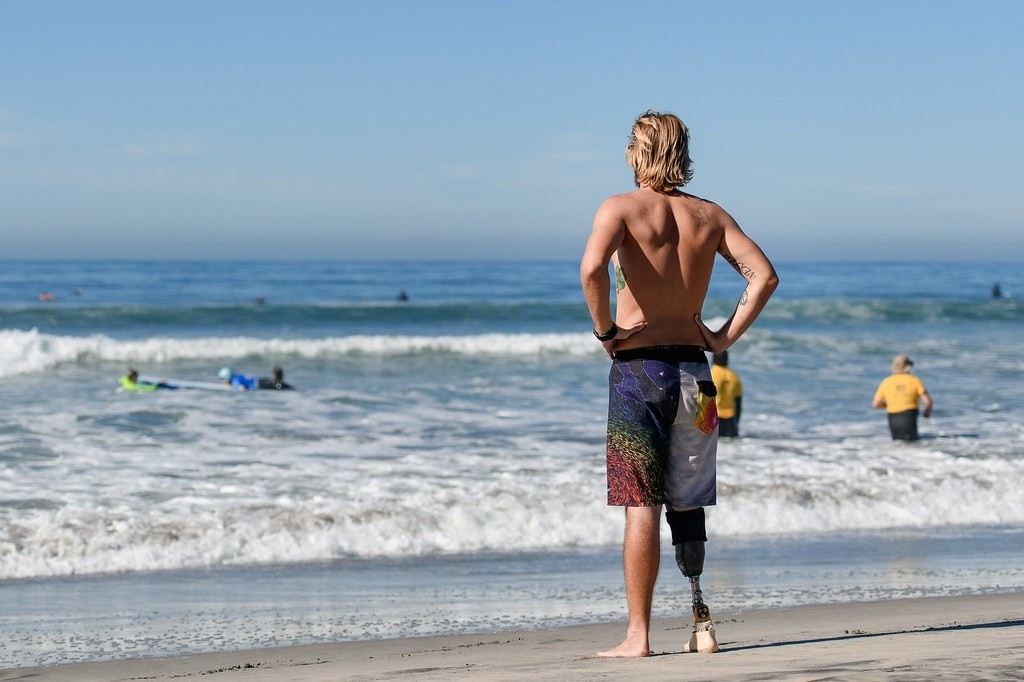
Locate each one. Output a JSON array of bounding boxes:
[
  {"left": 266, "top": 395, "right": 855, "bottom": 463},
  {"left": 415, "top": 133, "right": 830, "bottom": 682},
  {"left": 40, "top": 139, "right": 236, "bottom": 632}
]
[
  {"left": 115, "top": 369, "right": 165, "bottom": 392},
  {"left": 580, "top": 111, "right": 780, "bottom": 659},
  {"left": 872, "top": 355, "right": 931, "bottom": 440},
  {"left": 711, "top": 351, "right": 742, "bottom": 438},
  {"left": 219, "top": 367, "right": 290, "bottom": 391}
]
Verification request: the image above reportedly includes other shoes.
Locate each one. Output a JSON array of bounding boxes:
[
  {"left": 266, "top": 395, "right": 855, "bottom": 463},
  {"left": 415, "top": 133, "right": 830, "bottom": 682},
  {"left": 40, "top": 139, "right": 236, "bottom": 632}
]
[{"left": 682, "top": 628, "right": 718, "bottom": 657}]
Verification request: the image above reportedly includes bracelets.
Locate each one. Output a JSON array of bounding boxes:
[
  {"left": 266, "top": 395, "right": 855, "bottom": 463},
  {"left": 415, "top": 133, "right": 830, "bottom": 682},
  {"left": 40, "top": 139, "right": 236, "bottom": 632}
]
[{"left": 592, "top": 322, "right": 618, "bottom": 341}]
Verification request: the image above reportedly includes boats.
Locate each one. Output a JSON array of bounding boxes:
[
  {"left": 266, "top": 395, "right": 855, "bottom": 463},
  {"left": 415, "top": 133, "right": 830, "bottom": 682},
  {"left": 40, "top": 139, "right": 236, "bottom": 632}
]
[{"left": 135, "top": 375, "right": 234, "bottom": 393}]
